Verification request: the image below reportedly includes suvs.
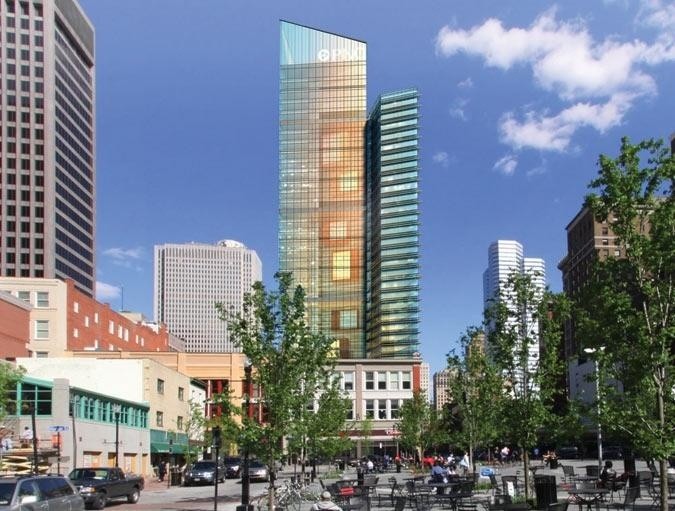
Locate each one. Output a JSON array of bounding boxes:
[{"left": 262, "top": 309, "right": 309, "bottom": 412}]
[{"left": 1, "top": 472, "right": 84, "bottom": 510}]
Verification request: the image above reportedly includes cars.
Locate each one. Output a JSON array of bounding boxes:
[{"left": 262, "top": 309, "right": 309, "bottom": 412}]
[
  {"left": 241, "top": 460, "right": 278, "bottom": 481},
  {"left": 184, "top": 460, "right": 226, "bottom": 484},
  {"left": 223, "top": 455, "right": 243, "bottom": 478}
]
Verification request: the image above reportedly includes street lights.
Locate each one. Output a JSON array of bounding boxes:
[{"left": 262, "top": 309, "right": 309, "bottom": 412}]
[
  {"left": 113, "top": 403, "right": 122, "bottom": 466},
  {"left": 583, "top": 343, "right": 605, "bottom": 469},
  {"left": 237, "top": 360, "right": 254, "bottom": 511}
]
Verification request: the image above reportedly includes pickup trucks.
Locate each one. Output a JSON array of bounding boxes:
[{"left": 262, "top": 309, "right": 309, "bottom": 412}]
[{"left": 70, "top": 468, "right": 144, "bottom": 511}]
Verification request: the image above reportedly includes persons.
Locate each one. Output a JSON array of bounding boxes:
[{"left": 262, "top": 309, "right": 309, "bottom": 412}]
[
  {"left": 326, "top": 447, "right": 474, "bottom": 482},
  {"left": 483, "top": 442, "right": 559, "bottom": 474},
  {"left": 22, "top": 426, "right": 32, "bottom": 441},
  {"left": 1, "top": 435, "right": 11, "bottom": 455}
]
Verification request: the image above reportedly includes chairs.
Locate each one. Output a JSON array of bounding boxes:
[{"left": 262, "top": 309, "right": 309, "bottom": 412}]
[
  {"left": 561, "top": 466, "right": 661, "bottom": 511},
  {"left": 317, "top": 468, "right": 522, "bottom": 510}
]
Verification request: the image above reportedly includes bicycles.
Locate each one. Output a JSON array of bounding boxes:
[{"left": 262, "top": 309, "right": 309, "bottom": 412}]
[{"left": 251, "top": 480, "right": 302, "bottom": 511}]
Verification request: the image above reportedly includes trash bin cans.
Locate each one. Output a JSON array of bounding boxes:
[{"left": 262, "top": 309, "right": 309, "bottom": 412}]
[
  {"left": 550, "top": 459, "right": 557, "bottom": 469},
  {"left": 534, "top": 474, "right": 557, "bottom": 505},
  {"left": 171, "top": 472, "right": 181, "bottom": 486}
]
